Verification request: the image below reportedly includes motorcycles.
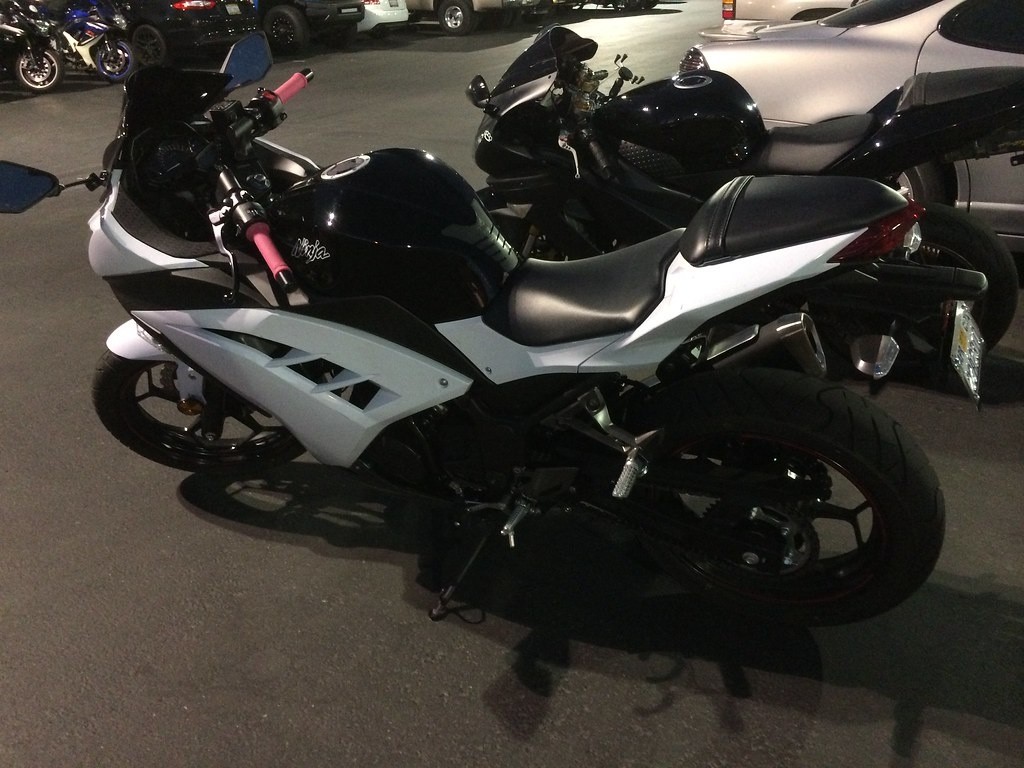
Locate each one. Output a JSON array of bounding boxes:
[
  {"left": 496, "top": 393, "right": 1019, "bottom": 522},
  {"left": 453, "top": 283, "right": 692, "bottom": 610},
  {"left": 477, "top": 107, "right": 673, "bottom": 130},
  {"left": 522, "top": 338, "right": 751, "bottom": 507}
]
[
  {"left": 2, "top": 0, "right": 140, "bottom": 85},
  {"left": 461, "top": 25, "right": 1021, "bottom": 386},
  {"left": 1, "top": 27, "right": 951, "bottom": 644}
]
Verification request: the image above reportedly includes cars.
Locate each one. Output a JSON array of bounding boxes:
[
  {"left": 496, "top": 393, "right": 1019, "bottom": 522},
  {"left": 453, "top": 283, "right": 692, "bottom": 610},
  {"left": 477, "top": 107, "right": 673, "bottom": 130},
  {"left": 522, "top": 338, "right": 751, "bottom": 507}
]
[
  {"left": 673, "top": 0, "right": 1024, "bottom": 251},
  {"left": 252, "top": 0, "right": 367, "bottom": 52},
  {"left": 405, "top": 0, "right": 542, "bottom": 38},
  {"left": 98, "top": 0, "right": 260, "bottom": 69},
  {"left": 341, "top": 0, "right": 410, "bottom": 36}
]
